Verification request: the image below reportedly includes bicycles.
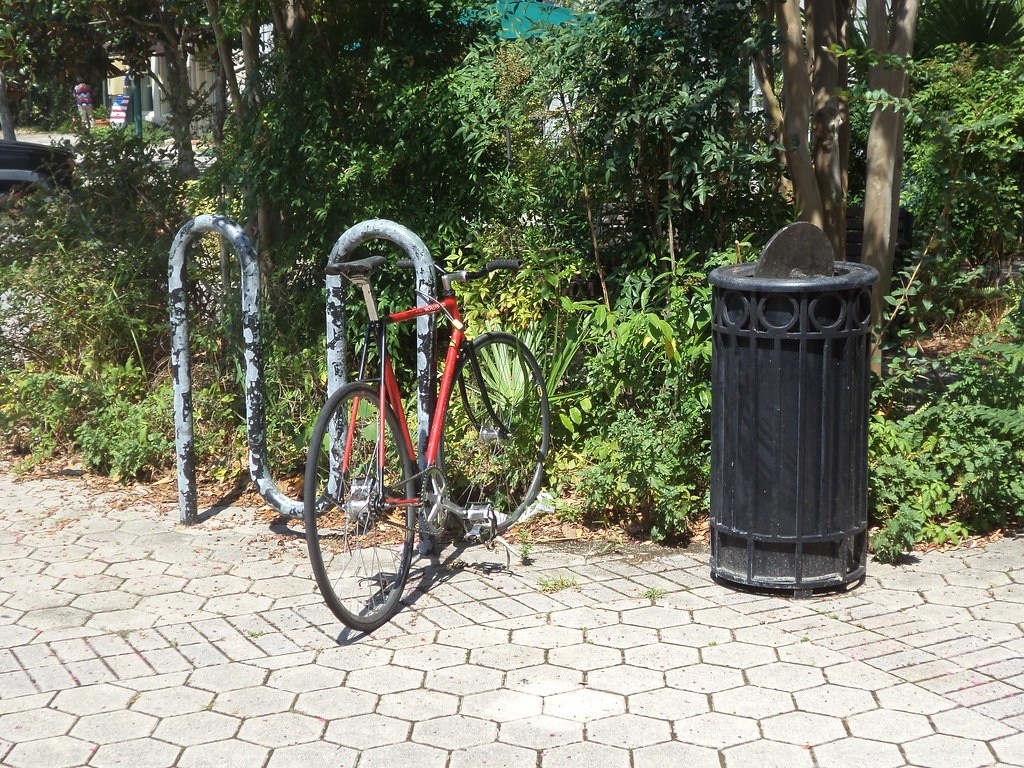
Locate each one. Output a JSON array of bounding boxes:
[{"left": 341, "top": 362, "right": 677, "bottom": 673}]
[{"left": 300, "top": 249, "right": 554, "bottom": 631}]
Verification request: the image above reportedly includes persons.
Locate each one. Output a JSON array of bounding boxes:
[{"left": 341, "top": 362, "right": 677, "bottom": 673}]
[{"left": 73, "top": 77, "right": 94, "bottom": 130}]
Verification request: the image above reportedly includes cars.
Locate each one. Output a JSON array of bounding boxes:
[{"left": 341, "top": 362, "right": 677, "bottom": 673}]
[{"left": 0, "top": 140, "right": 74, "bottom": 195}]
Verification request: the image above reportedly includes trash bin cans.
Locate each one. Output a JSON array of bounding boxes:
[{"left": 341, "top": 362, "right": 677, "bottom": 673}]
[{"left": 707, "top": 259, "right": 882, "bottom": 599}]
